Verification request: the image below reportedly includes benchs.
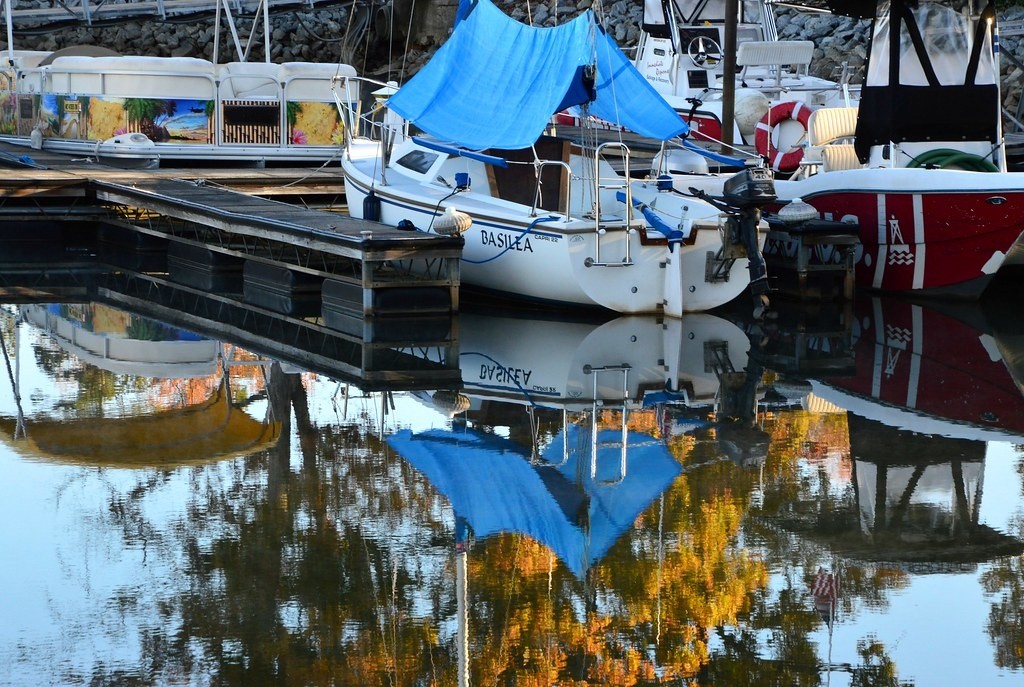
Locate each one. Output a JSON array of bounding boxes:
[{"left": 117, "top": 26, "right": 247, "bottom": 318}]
[{"left": 736, "top": 41, "right": 813, "bottom": 83}]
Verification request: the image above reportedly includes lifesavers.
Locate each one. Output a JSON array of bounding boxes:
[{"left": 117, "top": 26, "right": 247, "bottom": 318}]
[{"left": 755, "top": 102, "right": 815, "bottom": 173}]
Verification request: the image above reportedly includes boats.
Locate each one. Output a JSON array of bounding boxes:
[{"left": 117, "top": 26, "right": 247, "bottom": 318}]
[
  {"left": 0, "top": 0, "right": 362, "bottom": 168},
  {"left": 325, "top": 280, "right": 755, "bottom": 581},
  {"left": 340, "top": 0, "right": 776, "bottom": 312},
  {"left": 736, "top": 293, "right": 1023, "bottom": 573},
  {"left": 549, "top": 0, "right": 1024, "bottom": 296}
]
